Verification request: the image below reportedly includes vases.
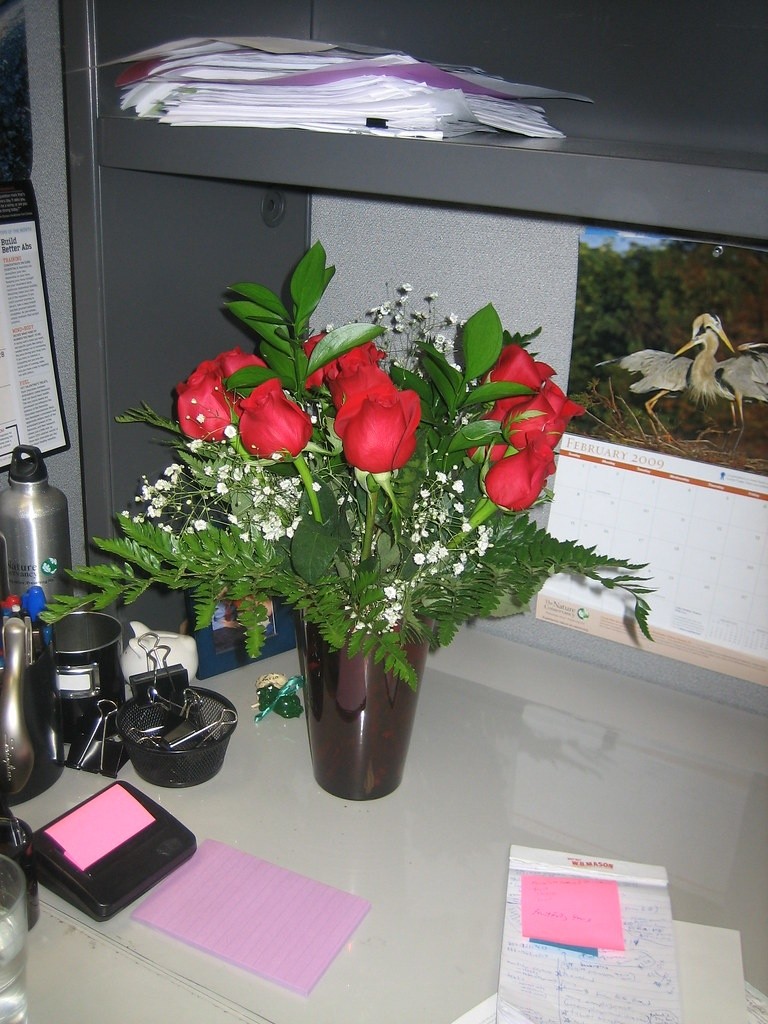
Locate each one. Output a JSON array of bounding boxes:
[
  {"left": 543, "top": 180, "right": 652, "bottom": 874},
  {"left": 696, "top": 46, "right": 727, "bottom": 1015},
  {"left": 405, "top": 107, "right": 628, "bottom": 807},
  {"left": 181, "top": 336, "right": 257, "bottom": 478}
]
[{"left": 288, "top": 603, "right": 437, "bottom": 801}]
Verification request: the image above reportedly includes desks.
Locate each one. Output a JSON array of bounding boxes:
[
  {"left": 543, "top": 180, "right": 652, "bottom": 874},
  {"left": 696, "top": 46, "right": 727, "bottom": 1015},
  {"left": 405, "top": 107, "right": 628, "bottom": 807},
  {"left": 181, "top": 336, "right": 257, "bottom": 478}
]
[{"left": 0, "top": 623, "right": 768, "bottom": 1024}]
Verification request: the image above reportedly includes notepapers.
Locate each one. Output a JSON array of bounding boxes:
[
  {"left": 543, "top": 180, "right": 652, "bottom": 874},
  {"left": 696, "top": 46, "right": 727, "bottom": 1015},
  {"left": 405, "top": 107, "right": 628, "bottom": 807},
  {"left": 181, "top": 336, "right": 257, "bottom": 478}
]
[{"left": 44, "top": 782, "right": 156, "bottom": 871}]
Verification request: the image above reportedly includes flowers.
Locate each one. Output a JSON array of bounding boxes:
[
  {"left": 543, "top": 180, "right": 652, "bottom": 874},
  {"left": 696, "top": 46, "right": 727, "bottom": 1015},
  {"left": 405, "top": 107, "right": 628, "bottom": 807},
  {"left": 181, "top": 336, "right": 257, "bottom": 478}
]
[{"left": 36, "top": 240, "right": 658, "bottom": 693}]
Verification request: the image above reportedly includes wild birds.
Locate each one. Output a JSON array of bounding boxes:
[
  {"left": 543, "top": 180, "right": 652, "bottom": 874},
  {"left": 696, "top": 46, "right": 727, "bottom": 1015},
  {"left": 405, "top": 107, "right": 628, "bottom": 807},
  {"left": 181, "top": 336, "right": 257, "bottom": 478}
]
[{"left": 593, "top": 312, "right": 768, "bottom": 455}]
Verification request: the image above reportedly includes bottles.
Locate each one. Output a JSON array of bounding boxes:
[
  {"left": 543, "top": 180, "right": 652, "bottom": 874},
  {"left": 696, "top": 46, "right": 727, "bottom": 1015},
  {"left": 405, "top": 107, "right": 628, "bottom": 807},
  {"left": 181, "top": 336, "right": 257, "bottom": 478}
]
[{"left": 0, "top": 444, "right": 74, "bottom": 606}]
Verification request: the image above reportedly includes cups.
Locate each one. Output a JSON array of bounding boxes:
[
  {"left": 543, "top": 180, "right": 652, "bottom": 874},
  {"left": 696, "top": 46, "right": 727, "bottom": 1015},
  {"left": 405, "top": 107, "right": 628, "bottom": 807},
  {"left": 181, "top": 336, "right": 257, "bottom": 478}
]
[
  {"left": 0, "top": 854, "right": 29, "bottom": 1024},
  {"left": 52, "top": 612, "right": 123, "bottom": 761},
  {"left": 0, "top": 630, "right": 65, "bottom": 805}
]
